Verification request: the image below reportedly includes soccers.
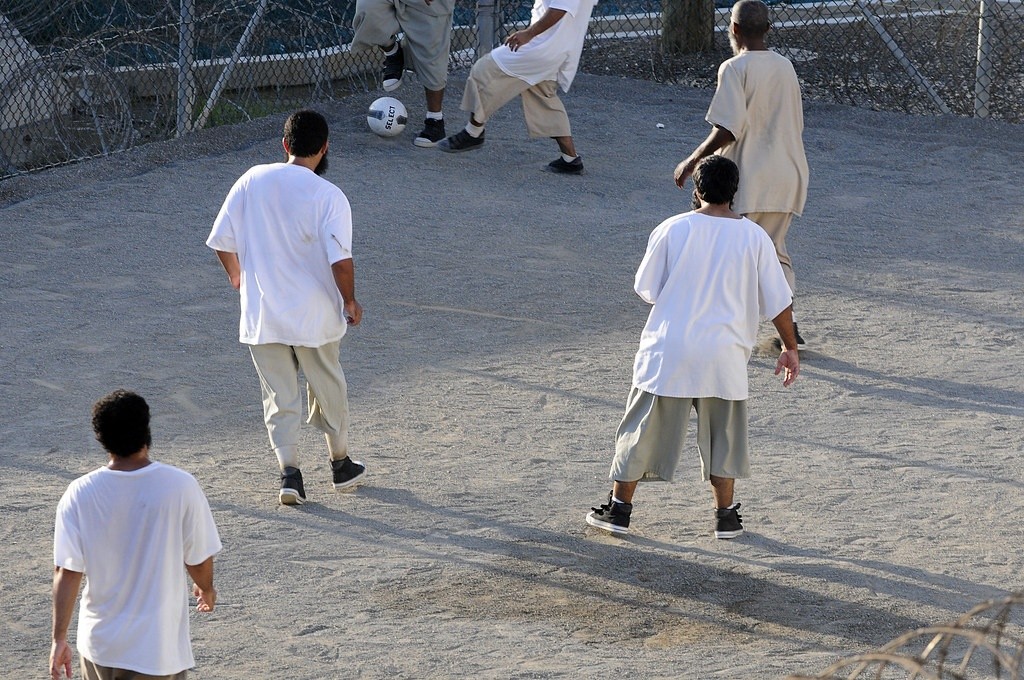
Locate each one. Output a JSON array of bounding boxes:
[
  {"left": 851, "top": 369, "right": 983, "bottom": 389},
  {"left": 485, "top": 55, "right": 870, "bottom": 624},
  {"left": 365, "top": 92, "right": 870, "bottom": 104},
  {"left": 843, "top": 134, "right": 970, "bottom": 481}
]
[{"left": 367, "top": 96, "right": 408, "bottom": 138}]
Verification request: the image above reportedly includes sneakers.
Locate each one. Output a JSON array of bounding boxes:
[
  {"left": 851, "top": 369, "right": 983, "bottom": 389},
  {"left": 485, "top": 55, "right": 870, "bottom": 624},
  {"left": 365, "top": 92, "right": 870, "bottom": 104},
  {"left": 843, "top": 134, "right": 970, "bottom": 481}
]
[
  {"left": 792, "top": 321, "right": 807, "bottom": 351},
  {"left": 586, "top": 490, "right": 633, "bottom": 535},
  {"left": 381, "top": 41, "right": 406, "bottom": 91},
  {"left": 278, "top": 465, "right": 307, "bottom": 505},
  {"left": 540, "top": 155, "right": 585, "bottom": 175},
  {"left": 713, "top": 502, "right": 744, "bottom": 539},
  {"left": 435, "top": 128, "right": 486, "bottom": 153},
  {"left": 328, "top": 455, "right": 368, "bottom": 490},
  {"left": 413, "top": 113, "right": 447, "bottom": 148}
]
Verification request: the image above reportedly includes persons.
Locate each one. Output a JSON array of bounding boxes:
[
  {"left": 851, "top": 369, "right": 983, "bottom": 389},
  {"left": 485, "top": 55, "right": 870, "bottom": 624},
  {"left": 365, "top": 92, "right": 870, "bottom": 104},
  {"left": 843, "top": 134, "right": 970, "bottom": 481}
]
[
  {"left": 205, "top": 110, "right": 365, "bottom": 505},
  {"left": 350, "top": 0, "right": 456, "bottom": 147},
  {"left": 586, "top": 154, "right": 801, "bottom": 538},
  {"left": 48, "top": 388, "right": 223, "bottom": 680},
  {"left": 436, "top": 0, "right": 600, "bottom": 175},
  {"left": 673, "top": 0, "right": 810, "bottom": 351}
]
